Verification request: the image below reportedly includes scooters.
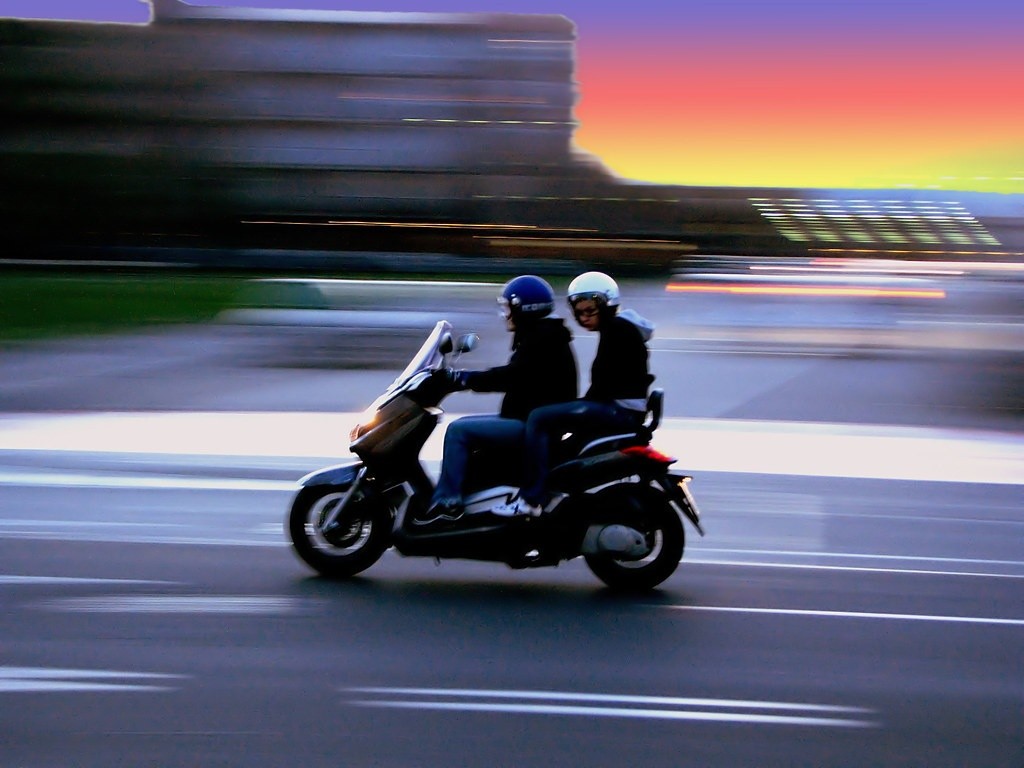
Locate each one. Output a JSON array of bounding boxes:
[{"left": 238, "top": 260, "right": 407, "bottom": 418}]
[{"left": 287, "top": 320, "right": 706, "bottom": 595}]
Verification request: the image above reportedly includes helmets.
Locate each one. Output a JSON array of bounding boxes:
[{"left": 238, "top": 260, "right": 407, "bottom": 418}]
[
  {"left": 496, "top": 275, "right": 556, "bottom": 322},
  {"left": 566, "top": 271, "right": 621, "bottom": 307}
]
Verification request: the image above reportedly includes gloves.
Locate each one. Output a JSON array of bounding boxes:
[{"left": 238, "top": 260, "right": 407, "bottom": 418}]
[{"left": 441, "top": 368, "right": 464, "bottom": 391}]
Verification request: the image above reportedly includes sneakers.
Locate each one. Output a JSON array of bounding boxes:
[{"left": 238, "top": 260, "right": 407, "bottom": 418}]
[
  {"left": 413, "top": 495, "right": 468, "bottom": 526},
  {"left": 489, "top": 494, "right": 542, "bottom": 518}
]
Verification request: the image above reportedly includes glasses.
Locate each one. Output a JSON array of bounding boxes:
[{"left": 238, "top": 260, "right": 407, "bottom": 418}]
[{"left": 573, "top": 306, "right": 600, "bottom": 317}]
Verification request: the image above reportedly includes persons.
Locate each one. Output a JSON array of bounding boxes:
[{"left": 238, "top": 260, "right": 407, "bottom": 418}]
[
  {"left": 411, "top": 275, "right": 578, "bottom": 525},
  {"left": 490, "top": 271, "right": 652, "bottom": 519}
]
